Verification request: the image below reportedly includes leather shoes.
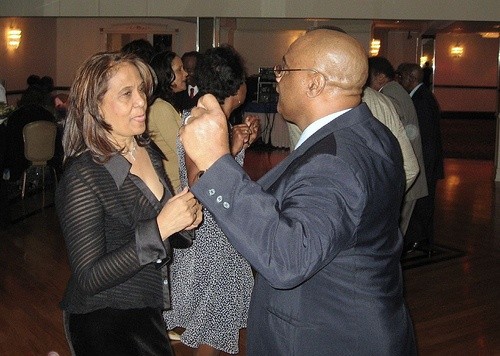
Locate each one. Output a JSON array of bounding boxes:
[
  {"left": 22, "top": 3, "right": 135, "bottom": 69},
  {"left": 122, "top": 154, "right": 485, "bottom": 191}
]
[{"left": 404, "top": 239, "right": 427, "bottom": 256}]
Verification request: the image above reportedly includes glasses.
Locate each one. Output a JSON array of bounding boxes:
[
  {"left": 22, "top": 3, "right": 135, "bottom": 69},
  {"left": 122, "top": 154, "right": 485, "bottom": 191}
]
[{"left": 273, "top": 65, "right": 328, "bottom": 82}]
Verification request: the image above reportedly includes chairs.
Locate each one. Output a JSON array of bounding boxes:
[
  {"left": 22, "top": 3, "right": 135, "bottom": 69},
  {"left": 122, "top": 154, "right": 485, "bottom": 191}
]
[{"left": 19, "top": 120, "right": 58, "bottom": 218}]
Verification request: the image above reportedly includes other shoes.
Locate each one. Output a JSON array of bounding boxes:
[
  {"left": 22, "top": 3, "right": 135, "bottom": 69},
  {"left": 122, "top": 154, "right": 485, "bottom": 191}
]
[{"left": 167, "top": 327, "right": 187, "bottom": 341}]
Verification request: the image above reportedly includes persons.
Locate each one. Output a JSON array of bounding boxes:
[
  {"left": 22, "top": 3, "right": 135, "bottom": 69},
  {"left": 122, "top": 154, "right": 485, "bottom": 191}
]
[
  {"left": 162, "top": 44, "right": 260, "bottom": 356},
  {"left": 121, "top": 37, "right": 207, "bottom": 196},
  {"left": 0, "top": 74, "right": 55, "bottom": 158},
  {"left": 286, "top": 25, "right": 420, "bottom": 194},
  {"left": 179, "top": 28, "right": 418, "bottom": 356},
  {"left": 397, "top": 62, "right": 443, "bottom": 252},
  {"left": 367, "top": 56, "right": 429, "bottom": 237},
  {"left": 46, "top": 52, "right": 203, "bottom": 356}
]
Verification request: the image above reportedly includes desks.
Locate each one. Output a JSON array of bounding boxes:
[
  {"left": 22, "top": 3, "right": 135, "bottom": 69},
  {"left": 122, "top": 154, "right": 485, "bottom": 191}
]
[{"left": 243, "top": 103, "right": 290, "bottom": 150}]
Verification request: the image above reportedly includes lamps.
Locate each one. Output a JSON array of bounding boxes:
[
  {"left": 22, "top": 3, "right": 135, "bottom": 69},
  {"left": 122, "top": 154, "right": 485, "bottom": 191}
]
[{"left": 7, "top": 25, "right": 23, "bottom": 46}]
